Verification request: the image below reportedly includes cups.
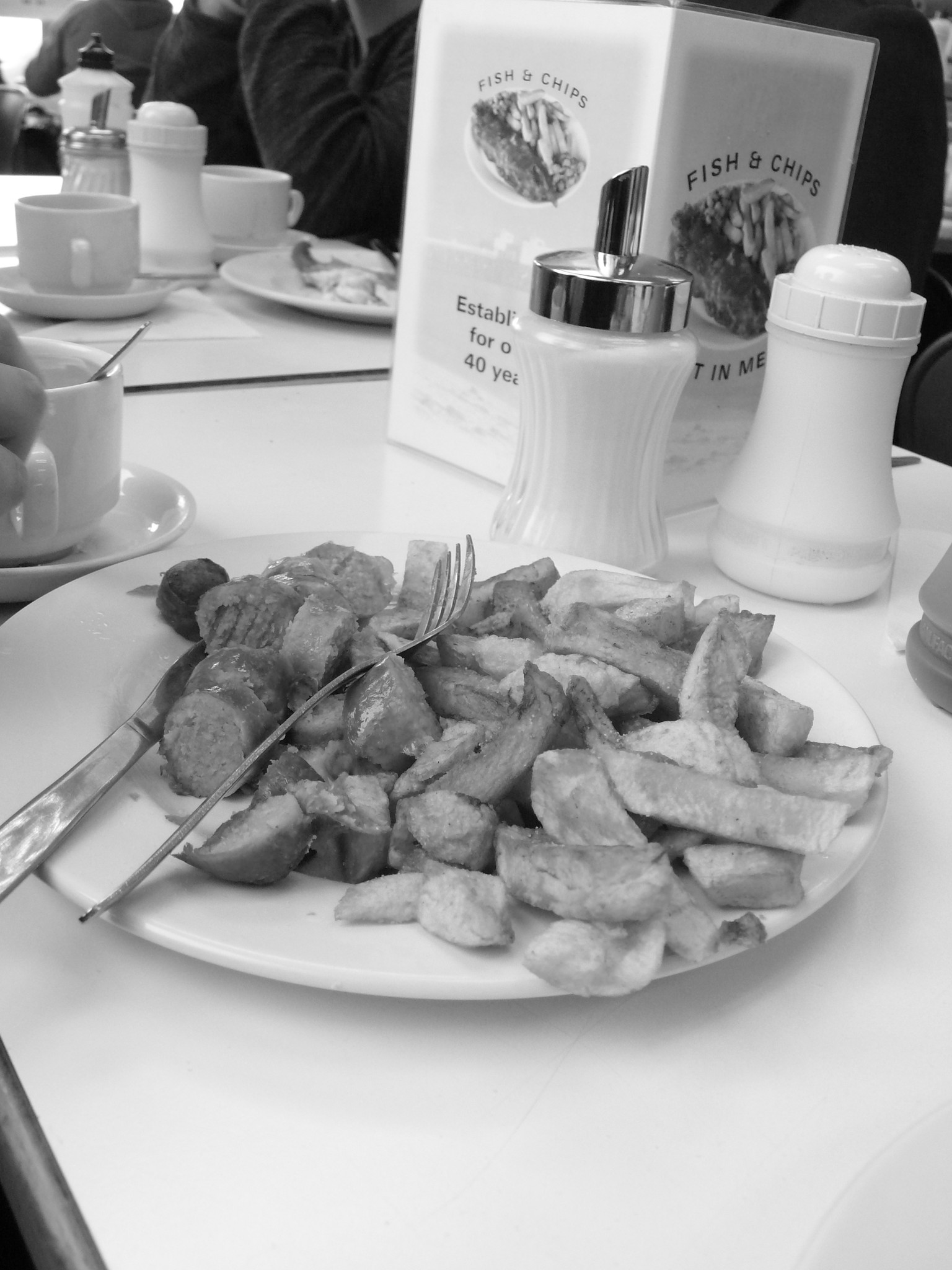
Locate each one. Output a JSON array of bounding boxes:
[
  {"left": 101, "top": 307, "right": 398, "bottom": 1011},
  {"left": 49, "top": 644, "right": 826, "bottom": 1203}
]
[
  {"left": 198, "top": 164, "right": 304, "bottom": 244},
  {"left": 14, "top": 192, "right": 141, "bottom": 295},
  {"left": 0, "top": 334, "right": 127, "bottom": 567}
]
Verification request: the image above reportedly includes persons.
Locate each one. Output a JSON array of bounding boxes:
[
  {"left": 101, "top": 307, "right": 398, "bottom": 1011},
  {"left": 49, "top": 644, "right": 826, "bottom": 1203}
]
[
  {"left": 25, "top": 1, "right": 173, "bottom": 113},
  {"left": 142, "top": 1, "right": 421, "bottom": 255}
]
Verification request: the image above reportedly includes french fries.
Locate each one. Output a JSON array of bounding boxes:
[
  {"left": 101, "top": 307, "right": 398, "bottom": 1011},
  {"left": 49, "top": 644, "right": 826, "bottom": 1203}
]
[{"left": 104, "top": 509, "right": 900, "bottom": 1012}]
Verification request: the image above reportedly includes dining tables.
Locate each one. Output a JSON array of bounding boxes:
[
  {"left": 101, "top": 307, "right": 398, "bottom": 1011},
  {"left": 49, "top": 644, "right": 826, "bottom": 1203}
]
[{"left": 1, "top": 174, "right": 952, "bottom": 1269}]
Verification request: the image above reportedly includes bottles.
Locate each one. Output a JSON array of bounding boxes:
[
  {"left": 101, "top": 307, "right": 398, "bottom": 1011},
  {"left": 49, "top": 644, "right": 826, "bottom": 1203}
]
[
  {"left": 710, "top": 245, "right": 926, "bottom": 608},
  {"left": 496, "top": 165, "right": 694, "bottom": 581},
  {"left": 128, "top": 101, "right": 215, "bottom": 288},
  {"left": 55, "top": 33, "right": 136, "bottom": 136}
]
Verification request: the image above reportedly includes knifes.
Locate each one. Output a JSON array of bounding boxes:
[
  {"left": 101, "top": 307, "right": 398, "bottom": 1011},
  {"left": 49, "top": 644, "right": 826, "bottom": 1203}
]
[{"left": 1, "top": 632, "right": 207, "bottom": 897}]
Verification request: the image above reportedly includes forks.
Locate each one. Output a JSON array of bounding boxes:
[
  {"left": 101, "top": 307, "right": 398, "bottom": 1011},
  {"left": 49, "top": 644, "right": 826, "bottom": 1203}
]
[{"left": 76, "top": 531, "right": 479, "bottom": 929}]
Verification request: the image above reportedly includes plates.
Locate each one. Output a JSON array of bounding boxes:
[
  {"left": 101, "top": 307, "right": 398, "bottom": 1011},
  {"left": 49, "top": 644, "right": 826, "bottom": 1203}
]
[
  {"left": 207, "top": 228, "right": 322, "bottom": 265},
  {"left": 0, "top": 265, "right": 171, "bottom": 320},
  {"left": 0, "top": 462, "right": 198, "bottom": 605},
  {"left": 220, "top": 248, "right": 401, "bottom": 327},
  {"left": 0, "top": 532, "right": 893, "bottom": 998}
]
